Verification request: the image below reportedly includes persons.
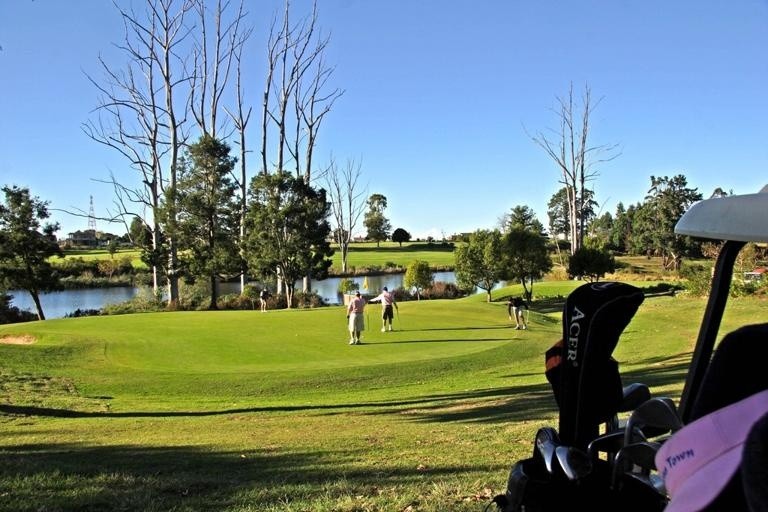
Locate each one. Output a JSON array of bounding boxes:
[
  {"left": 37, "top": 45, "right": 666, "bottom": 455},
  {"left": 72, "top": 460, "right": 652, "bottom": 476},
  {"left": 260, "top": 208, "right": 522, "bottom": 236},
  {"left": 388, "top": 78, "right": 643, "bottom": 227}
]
[
  {"left": 346, "top": 286, "right": 398, "bottom": 345},
  {"left": 507, "top": 296, "right": 529, "bottom": 330},
  {"left": 259, "top": 287, "right": 269, "bottom": 312}
]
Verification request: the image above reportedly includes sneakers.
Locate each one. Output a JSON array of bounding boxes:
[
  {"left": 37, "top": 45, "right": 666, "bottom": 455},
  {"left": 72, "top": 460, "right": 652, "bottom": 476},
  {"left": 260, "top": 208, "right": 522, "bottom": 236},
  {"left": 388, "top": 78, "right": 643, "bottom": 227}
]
[
  {"left": 349, "top": 339, "right": 361, "bottom": 345},
  {"left": 381, "top": 325, "right": 393, "bottom": 333},
  {"left": 514, "top": 324, "right": 527, "bottom": 330}
]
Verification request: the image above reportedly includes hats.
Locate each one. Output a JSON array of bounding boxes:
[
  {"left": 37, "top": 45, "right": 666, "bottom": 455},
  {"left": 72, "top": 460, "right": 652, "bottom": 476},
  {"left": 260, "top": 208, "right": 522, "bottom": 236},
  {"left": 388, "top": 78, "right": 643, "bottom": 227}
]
[
  {"left": 653, "top": 389, "right": 767, "bottom": 512},
  {"left": 354, "top": 291, "right": 360, "bottom": 296}
]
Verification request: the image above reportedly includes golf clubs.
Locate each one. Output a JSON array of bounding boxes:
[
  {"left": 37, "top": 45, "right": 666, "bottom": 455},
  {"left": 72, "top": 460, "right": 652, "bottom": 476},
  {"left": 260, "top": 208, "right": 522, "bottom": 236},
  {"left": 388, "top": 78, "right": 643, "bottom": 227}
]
[
  {"left": 533, "top": 426, "right": 627, "bottom": 481},
  {"left": 527, "top": 312, "right": 530, "bottom": 324},
  {"left": 605, "top": 382, "right": 681, "bottom": 507}
]
